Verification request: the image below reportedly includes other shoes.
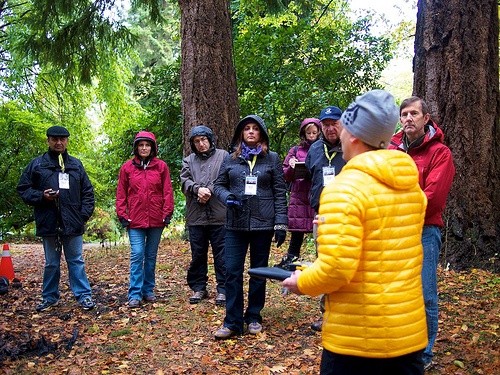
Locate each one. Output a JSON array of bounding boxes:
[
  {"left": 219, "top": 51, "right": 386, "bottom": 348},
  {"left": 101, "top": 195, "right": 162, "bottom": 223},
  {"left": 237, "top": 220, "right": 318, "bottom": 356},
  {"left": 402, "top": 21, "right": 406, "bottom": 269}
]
[
  {"left": 311, "top": 318, "right": 323, "bottom": 331},
  {"left": 274, "top": 257, "right": 296, "bottom": 272},
  {"left": 215, "top": 326, "right": 233, "bottom": 340},
  {"left": 189, "top": 290, "right": 208, "bottom": 303},
  {"left": 215, "top": 294, "right": 227, "bottom": 306},
  {"left": 129, "top": 299, "right": 141, "bottom": 308},
  {"left": 248, "top": 321, "right": 262, "bottom": 334},
  {"left": 144, "top": 295, "right": 156, "bottom": 302},
  {"left": 423, "top": 360, "right": 433, "bottom": 371}
]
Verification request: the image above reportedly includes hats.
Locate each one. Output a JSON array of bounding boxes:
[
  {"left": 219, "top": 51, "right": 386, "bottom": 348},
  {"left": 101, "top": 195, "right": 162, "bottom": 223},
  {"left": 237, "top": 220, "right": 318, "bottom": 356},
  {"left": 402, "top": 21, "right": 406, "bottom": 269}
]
[
  {"left": 46, "top": 126, "right": 70, "bottom": 137},
  {"left": 320, "top": 107, "right": 342, "bottom": 122},
  {"left": 341, "top": 89, "right": 400, "bottom": 148}
]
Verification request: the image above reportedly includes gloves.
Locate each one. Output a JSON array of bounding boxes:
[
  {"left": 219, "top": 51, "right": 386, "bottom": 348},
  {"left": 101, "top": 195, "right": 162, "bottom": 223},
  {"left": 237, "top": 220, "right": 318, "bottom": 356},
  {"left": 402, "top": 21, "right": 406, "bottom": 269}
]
[
  {"left": 120, "top": 218, "right": 129, "bottom": 227},
  {"left": 164, "top": 215, "right": 170, "bottom": 224},
  {"left": 275, "top": 230, "right": 286, "bottom": 248},
  {"left": 226, "top": 196, "right": 238, "bottom": 212}
]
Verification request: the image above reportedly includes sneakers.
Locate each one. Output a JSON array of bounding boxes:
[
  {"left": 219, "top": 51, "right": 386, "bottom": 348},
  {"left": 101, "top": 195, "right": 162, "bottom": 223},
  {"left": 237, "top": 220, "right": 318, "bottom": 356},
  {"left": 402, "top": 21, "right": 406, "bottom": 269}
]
[
  {"left": 80, "top": 297, "right": 94, "bottom": 311},
  {"left": 36, "top": 300, "right": 60, "bottom": 312}
]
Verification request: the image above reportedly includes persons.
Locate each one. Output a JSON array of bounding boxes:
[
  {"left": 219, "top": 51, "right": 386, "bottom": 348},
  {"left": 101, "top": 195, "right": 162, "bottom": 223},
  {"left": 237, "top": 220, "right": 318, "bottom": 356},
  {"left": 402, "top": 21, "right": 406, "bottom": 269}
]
[
  {"left": 388, "top": 96, "right": 455, "bottom": 371},
  {"left": 17, "top": 127, "right": 95, "bottom": 310},
  {"left": 213, "top": 115, "right": 288, "bottom": 339},
  {"left": 179, "top": 126, "right": 229, "bottom": 305},
  {"left": 274, "top": 118, "right": 324, "bottom": 271},
  {"left": 116, "top": 132, "right": 174, "bottom": 310},
  {"left": 281, "top": 89, "right": 427, "bottom": 375},
  {"left": 304, "top": 106, "right": 343, "bottom": 331}
]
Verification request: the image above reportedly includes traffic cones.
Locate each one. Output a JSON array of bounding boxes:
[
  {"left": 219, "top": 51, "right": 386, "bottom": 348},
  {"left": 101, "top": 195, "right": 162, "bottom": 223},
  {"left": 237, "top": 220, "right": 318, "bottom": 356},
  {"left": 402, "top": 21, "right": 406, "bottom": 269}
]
[{"left": 0, "top": 243, "right": 18, "bottom": 285}]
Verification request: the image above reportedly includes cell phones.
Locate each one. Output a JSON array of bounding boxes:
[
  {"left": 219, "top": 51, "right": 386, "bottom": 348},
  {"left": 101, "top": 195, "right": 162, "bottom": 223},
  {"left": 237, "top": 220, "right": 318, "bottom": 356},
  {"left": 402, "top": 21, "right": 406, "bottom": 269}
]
[{"left": 49, "top": 190, "right": 56, "bottom": 195}]
